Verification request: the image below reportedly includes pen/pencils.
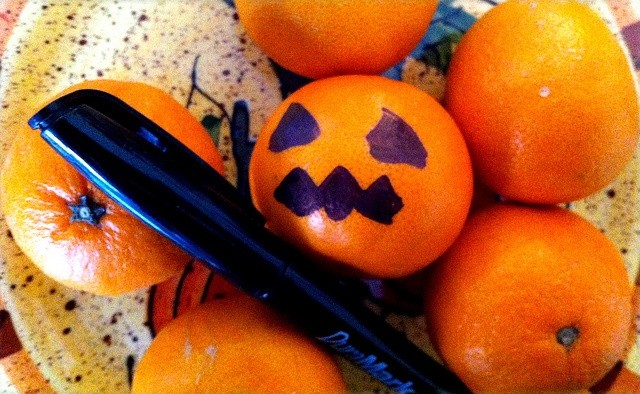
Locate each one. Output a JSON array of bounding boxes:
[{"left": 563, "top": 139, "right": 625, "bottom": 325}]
[{"left": 27, "top": 88, "right": 472, "bottom": 393}]
